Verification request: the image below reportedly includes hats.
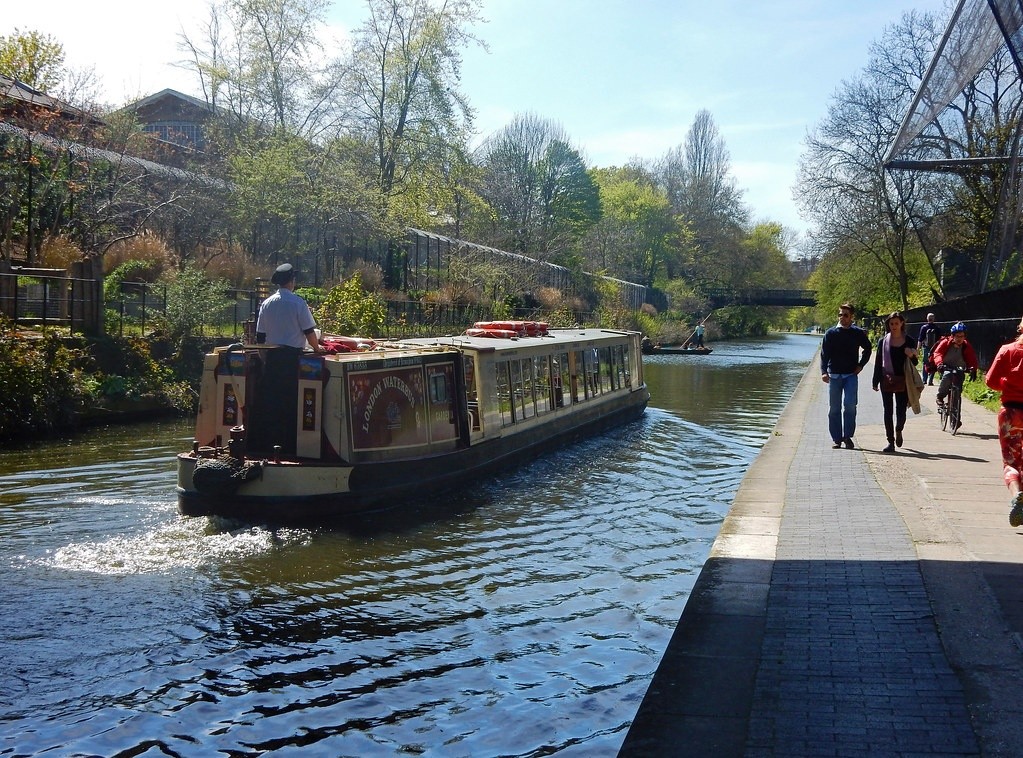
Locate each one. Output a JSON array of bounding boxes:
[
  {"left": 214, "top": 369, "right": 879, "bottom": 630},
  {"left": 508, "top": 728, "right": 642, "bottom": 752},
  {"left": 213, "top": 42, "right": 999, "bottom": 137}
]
[{"left": 270, "top": 262, "right": 299, "bottom": 285}]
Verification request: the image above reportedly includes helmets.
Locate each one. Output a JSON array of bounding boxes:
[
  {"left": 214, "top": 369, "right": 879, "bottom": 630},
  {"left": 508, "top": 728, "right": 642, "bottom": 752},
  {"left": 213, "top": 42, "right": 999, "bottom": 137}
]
[{"left": 951, "top": 323, "right": 967, "bottom": 334}]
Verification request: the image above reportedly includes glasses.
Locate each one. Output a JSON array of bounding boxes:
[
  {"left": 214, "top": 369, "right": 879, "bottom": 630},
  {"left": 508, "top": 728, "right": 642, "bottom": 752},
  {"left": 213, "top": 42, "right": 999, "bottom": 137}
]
[{"left": 838, "top": 313, "right": 852, "bottom": 318}]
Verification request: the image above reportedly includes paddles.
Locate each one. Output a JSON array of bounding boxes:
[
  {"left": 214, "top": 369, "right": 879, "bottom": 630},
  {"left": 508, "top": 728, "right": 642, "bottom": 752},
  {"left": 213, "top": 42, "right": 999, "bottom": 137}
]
[{"left": 679, "top": 313, "right": 712, "bottom": 349}]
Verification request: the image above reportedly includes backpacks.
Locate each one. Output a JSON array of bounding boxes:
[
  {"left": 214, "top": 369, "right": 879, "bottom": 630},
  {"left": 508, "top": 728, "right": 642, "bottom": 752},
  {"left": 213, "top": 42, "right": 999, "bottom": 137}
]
[{"left": 927, "top": 337, "right": 967, "bottom": 372}]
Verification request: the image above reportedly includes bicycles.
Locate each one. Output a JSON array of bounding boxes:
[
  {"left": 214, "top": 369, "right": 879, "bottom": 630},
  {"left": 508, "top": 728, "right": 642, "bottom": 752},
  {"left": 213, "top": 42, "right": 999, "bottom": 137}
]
[{"left": 937, "top": 367, "right": 972, "bottom": 435}]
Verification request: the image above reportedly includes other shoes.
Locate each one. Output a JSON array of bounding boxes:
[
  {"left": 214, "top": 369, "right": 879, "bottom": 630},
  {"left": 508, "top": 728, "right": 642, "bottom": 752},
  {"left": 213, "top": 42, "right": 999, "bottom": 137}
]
[
  {"left": 1009, "top": 491, "right": 1023, "bottom": 527},
  {"left": 844, "top": 438, "right": 855, "bottom": 449},
  {"left": 896, "top": 428, "right": 904, "bottom": 447},
  {"left": 929, "top": 383, "right": 934, "bottom": 386},
  {"left": 954, "top": 421, "right": 962, "bottom": 430},
  {"left": 832, "top": 443, "right": 841, "bottom": 448},
  {"left": 936, "top": 398, "right": 943, "bottom": 406},
  {"left": 884, "top": 445, "right": 895, "bottom": 453}
]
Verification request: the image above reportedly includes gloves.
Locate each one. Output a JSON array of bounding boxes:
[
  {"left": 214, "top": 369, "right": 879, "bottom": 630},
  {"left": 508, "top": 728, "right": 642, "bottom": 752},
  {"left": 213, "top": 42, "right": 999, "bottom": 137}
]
[
  {"left": 969, "top": 371, "right": 977, "bottom": 382},
  {"left": 937, "top": 364, "right": 946, "bottom": 373}
]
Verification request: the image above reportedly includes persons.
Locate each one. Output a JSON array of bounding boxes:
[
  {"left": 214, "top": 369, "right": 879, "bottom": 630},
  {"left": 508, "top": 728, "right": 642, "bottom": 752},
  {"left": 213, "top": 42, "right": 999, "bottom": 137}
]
[
  {"left": 640, "top": 335, "right": 658, "bottom": 350},
  {"left": 694, "top": 321, "right": 706, "bottom": 348},
  {"left": 933, "top": 321, "right": 979, "bottom": 431},
  {"left": 872, "top": 311, "right": 925, "bottom": 455},
  {"left": 816, "top": 326, "right": 826, "bottom": 334},
  {"left": 820, "top": 302, "right": 872, "bottom": 450},
  {"left": 916, "top": 313, "right": 942, "bottom": 386},
  {"left": 654, "top": 341, "right": 664, "bottom": 349},
  {"left": 984, "top": 315, "right": 1023, "bottom": 528},
  {"left": 687, "top": 342, "right": 696, "bottom": 350},
  {"left": 253, "top": 262, "right": 327, "bottom": 461}
]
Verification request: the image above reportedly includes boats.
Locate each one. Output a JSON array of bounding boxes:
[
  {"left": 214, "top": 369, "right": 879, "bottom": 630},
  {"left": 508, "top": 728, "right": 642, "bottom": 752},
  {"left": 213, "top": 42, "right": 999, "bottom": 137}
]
[
  {"left": 177, "top": 319, "right": 651, "bottom": 524},
  {"left": 641, "top": 346, "right": 713, "bottom": 355}
]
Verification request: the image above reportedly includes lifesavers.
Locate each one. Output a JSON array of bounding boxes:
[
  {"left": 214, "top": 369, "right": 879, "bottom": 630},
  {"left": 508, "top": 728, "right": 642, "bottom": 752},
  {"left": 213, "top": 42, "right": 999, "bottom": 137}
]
[
  {"left": 466, "top": 320, "right": 548, "bottom": 338},
  {"left": 323, "top": 336, "right": 374, "bottom": 348}
]
